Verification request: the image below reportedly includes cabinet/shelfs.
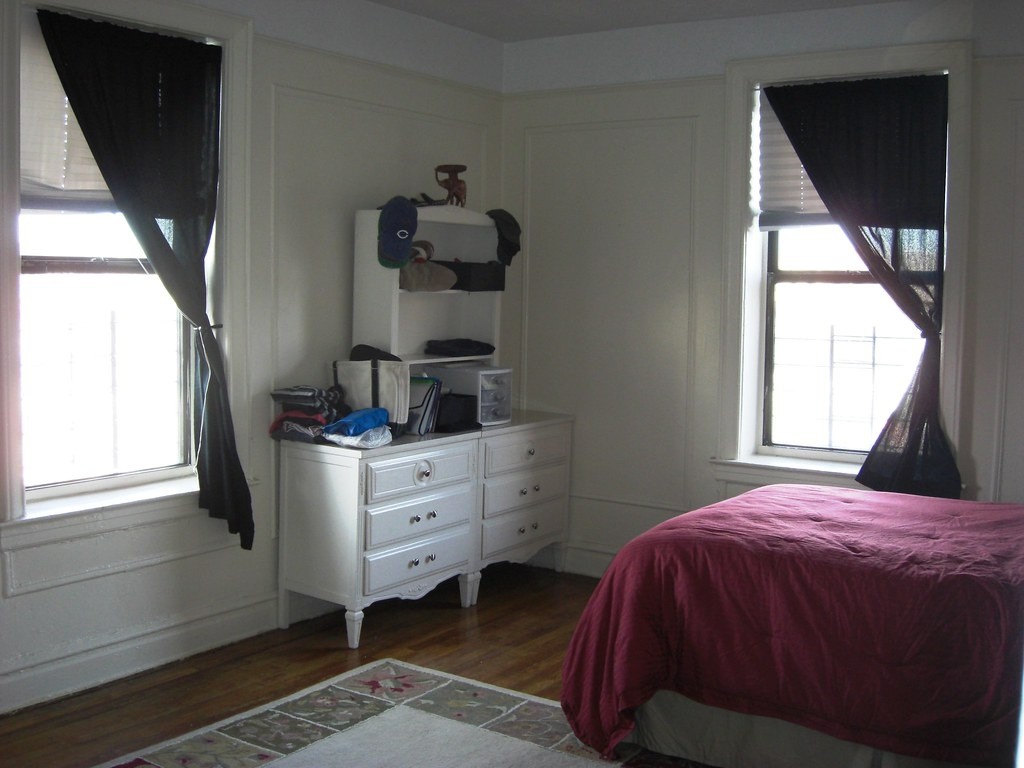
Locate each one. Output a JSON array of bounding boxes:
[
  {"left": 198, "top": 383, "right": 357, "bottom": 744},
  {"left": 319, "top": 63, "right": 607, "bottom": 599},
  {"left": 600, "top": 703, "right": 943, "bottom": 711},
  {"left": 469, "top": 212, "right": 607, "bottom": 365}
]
[
  {"left": 350, "top": 204, "right": 503, "bottom": 407},
  {"left": 277, "top": 408, "right": 577, "bottom": 649},
  {"left": 424, "top": 366, "right": 513, "bottom": 426}
]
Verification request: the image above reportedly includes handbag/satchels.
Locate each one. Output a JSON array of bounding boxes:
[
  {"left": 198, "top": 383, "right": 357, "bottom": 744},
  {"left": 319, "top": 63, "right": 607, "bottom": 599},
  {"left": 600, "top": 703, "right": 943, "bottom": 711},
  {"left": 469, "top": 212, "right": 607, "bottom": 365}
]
[{"left": 308, "top": 405, "right": 392, "bottom": 450}]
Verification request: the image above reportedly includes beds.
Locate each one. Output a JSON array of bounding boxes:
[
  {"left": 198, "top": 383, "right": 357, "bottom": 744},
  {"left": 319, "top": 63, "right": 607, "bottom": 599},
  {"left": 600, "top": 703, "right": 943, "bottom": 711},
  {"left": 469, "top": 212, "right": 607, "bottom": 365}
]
[{"left": 559, "top": 484, "right": 1024, "bottom": 768}]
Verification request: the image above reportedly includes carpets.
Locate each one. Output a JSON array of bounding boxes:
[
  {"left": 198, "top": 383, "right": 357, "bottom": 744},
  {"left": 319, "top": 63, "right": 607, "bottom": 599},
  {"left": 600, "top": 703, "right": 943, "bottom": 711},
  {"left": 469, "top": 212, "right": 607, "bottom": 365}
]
[{"left": 95, "top": 658, "right": 722, "bottom": 768}]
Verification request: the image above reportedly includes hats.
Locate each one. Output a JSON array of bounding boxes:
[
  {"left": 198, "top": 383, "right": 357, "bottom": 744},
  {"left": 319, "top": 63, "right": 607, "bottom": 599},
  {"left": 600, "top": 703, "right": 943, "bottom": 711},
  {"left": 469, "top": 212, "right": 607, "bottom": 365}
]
[
  {"left": 486, "top": 208, "right": 522, "bottom": 265},
  {"left": 376, "top": 196, "right": 419, "bottom": 269}
]
